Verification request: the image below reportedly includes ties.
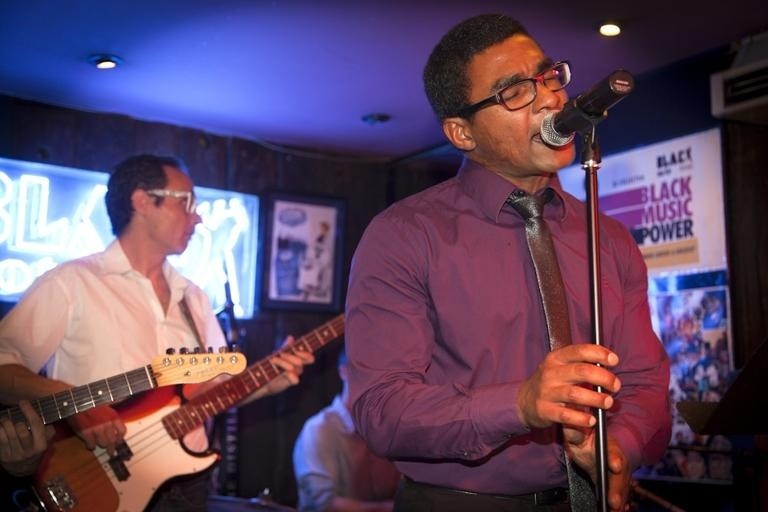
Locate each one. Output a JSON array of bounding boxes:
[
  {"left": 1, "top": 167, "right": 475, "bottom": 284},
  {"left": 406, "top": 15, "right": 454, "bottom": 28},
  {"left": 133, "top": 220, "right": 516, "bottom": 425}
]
[{"left": 505, "top": 188, "right": 597, "bottom": 512}]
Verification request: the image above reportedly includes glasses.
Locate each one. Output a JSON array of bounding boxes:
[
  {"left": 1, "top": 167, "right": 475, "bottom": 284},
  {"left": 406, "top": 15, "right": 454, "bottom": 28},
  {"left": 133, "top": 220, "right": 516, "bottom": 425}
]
[
  {"left": 147, "top": 189, "right": 197, "bottom": 215},
  {"left": 456, "top": 59, "right": 573, "bottom": 117}
]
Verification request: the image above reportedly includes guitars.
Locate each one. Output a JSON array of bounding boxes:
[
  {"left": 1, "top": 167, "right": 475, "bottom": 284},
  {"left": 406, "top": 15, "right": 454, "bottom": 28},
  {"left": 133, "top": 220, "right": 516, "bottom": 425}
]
[
  {"left": 0, "top": 345, "right": 246, "bottom": 432},
  {"left": 0, "top": 312, "right": 346, "bottom": 511}
]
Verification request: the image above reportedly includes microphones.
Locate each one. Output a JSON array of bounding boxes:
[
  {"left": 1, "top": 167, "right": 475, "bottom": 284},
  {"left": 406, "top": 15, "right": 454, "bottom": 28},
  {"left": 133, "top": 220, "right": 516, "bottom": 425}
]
[
  {"left": 541, "top": 70, "right": 634, "bottom": 149},
  {"left": 223, "top": 254, "right": 239, "bottom": 343}
]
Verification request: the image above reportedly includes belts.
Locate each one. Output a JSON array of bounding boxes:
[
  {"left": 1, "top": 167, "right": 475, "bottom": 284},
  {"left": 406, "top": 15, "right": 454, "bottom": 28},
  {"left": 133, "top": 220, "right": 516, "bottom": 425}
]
[{"left": 414, "top": 485, "right": 571, "bottom": 512}]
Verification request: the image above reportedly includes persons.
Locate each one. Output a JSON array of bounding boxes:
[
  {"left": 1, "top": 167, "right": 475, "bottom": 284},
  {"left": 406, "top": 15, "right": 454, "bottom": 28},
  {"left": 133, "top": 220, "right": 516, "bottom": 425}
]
[
  {"left": 345, "top": 13, "right": 671, "bottom": 512},
  {"left": 1, "top": 154, "right": 315, "bottom": 511},
  {"left": 292, "top": 347, "right": 395, "bottom": 512}
]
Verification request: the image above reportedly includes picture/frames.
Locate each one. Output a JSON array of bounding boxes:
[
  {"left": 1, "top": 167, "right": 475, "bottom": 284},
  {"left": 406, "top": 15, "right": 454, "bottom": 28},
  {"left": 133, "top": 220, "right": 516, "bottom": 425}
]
[{"left": 260, "top": 193, "right": 345, "bottom": 314}]
